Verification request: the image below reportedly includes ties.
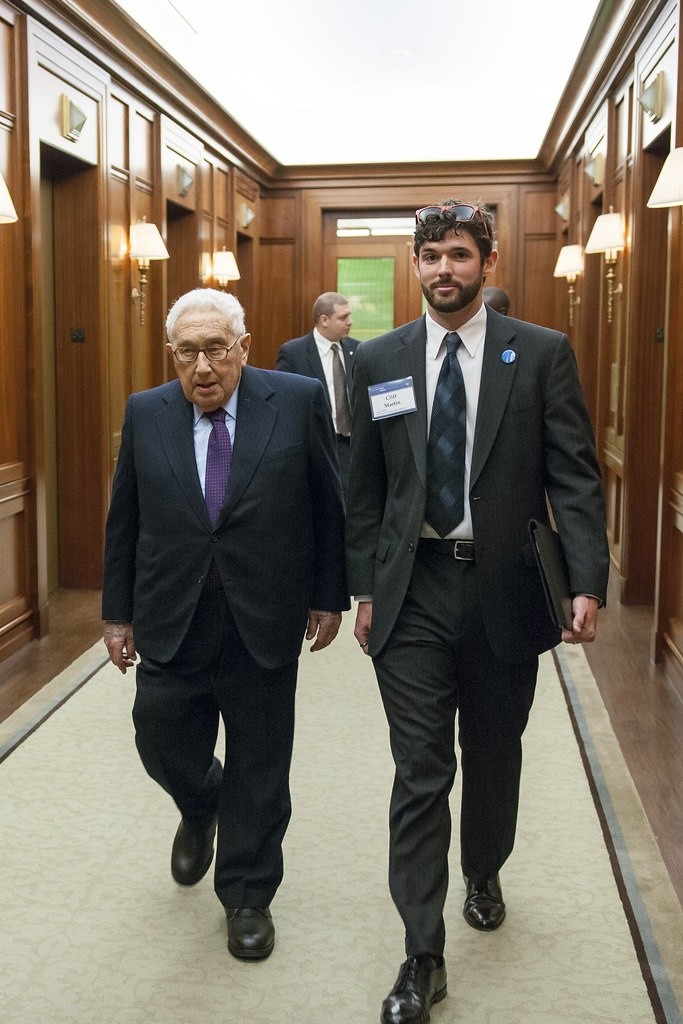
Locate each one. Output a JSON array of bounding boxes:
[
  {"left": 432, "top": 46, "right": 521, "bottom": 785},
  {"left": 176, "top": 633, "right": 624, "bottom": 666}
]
[
  {"left": 422, "top": 334, "right": 467, "bottom": 538},
  {"left": 205, "top": 407, "right": 232, "bottom": 527},
  {"left": 330, "top": 343, "right": 351, "bottom": 437}
]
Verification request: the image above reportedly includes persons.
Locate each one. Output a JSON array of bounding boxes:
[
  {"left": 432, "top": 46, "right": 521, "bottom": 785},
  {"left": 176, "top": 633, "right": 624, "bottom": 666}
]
[
  {"left": 275, "top": 291, "right": 362, "bottom": 515},
  {"left": 99, "top": 289, "right": 352, "bottom": 962},
  {"left": 341, "top": 199, "right": 611, "bottom": 1024},
  {"left": 482, "top": 286, "right": 509, "bottom": 316}
]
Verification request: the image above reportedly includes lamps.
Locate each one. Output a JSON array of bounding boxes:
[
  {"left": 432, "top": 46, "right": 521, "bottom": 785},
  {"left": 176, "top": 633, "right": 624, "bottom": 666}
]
[
  {"left": 212, "top": 248, "right": 241, "bottom": 293},
  {"left": 131, "top": 215, "right": 171, "bottom": 325},
  {"left": 553, "top": 245, "right": 584, "bottom": 326},
  {"left": 647, "top": 147, "right": 683, "bottom": 208},
  {"left": 586, "top": 204, "right": 628, "bottom": 321}
]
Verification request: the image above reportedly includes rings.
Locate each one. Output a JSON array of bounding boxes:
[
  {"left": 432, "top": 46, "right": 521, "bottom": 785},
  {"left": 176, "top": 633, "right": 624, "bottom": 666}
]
[{"left": 360, "top": 643, "right": 368, "bottom": 648}]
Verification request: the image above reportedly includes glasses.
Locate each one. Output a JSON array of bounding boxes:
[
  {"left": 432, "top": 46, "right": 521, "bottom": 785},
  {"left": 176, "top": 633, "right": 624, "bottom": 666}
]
[
  {"left": 415, "top": 204, "right": 489, "bottom": 237},
  {"left": 171, "top": 331, "right": 241, "bottom": 361}
]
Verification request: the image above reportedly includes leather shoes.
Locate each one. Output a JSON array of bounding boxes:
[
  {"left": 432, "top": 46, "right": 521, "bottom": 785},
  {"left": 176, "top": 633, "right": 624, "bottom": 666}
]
[
  {"left": 224, "top": 904, "right": 275, "bottom": 959},
  {"left": 380, "top": 952, "right": 448, "bottom": 1024},
  {"left": 462, "top": 871, "right": 506, "bottom": 932},
  {"left": 170, "top": 755, "right": 223, "bottom": 885}
]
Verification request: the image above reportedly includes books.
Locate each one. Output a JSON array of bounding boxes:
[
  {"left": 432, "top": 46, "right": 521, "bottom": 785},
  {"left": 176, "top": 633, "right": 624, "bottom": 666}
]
[{"left": 527, "top": 519, "right": 579, "bottom": 633}]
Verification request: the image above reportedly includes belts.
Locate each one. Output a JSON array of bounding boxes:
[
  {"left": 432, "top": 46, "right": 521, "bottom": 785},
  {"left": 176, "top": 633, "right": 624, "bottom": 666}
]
[{"left": 417, "top": 538, "right": 476, "bottom": 561}]
